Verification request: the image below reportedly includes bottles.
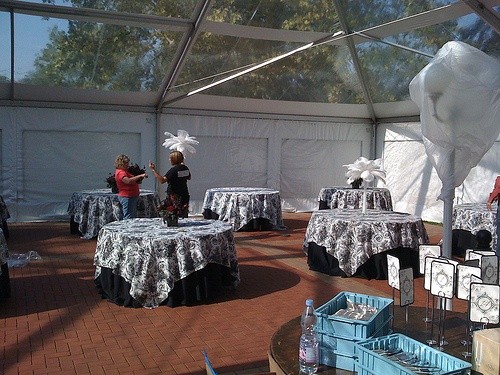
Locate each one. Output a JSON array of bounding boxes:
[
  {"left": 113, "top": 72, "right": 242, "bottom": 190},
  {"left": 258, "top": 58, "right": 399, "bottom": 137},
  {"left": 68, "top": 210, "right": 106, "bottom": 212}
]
[{"left": 299, "top": 299, "right": 319, "bottom": 374}]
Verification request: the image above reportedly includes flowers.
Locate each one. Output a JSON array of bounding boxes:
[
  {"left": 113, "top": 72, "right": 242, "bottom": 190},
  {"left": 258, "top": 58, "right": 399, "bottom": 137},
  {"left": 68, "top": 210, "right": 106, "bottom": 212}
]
[
  {"left": 104, "top": 163, "right": 147, "bottom": 189},
  {"left": 156, "top": 193, "right": 190, "bottom": 223}
]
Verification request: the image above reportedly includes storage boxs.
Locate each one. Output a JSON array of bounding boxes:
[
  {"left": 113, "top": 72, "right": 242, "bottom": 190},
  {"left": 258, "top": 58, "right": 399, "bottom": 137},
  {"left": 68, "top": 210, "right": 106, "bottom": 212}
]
[
  {"left": 313, "top": 290, "right": 394, "bottom": 373},
  {"left": 354, "top": 333, "right": 472, "bottom": 375}
]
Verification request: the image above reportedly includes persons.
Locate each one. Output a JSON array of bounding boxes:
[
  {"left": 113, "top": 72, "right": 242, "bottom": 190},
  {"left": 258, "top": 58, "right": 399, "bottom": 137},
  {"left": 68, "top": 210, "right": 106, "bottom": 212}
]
[
  {"left": 114, "top": 155, "right": 148, "bottom": 219},
  {"left": 487, "top": 176, "right": 500, "bottom": 257},
  {"left": 149, "top": 151, "right": 191, "bottom": 219}
]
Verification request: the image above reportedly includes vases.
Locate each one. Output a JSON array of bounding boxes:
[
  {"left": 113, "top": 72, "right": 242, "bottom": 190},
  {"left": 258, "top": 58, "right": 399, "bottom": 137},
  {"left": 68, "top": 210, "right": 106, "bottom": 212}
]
[
  {"left": 168, "top": 215, "right": 179, "bottom": 227},
  {"left": 111, "top": 186, "right": 119, "bottom": 194}
]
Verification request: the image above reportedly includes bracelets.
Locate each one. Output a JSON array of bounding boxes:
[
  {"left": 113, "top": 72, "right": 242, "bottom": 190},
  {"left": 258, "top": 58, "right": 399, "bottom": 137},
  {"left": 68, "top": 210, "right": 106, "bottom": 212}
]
[{"left": 152, "top": 170, "right": 154, "bottom": 172}]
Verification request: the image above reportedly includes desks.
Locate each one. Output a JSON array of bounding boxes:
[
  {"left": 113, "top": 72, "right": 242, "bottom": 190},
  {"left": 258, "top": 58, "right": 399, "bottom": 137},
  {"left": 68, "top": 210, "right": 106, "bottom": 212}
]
[
  {"left": 67, "top": 189, "right": 161, "bottom": 240},
  {"left": 437, "top": 203, "right": 498, "bottom": 258},
  {"left": 317, "top": 186, "right": 393, "bottom": 211},
  {"left": 93, "top": 218, "right": 240, "bottom": 309},
  {"left": 201, "top": 187, "right": 288, "bottom": 231},
  {"left": 302, "top": 207, "right": 430, "bottom": 280},
  {"left": 267, "top": 306, "right": 500, "bottom": 375}
]
